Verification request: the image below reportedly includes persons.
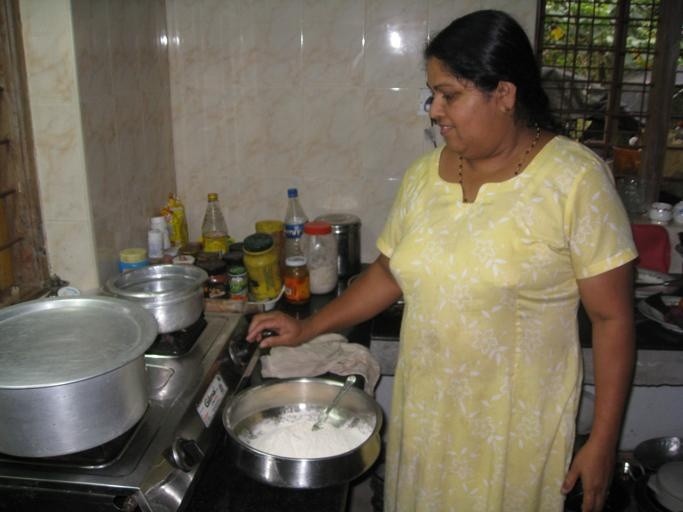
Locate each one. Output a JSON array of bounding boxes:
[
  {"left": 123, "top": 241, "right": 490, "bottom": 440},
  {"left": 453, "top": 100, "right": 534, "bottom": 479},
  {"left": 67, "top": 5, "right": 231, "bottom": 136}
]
[{"left": 246, "top": 10, "right": 640, "bottom": 512}]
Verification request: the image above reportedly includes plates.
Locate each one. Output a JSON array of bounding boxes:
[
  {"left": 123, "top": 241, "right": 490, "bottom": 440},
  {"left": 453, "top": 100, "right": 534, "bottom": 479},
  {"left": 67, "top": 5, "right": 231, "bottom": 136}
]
[{"left": 632, "top": 266, "right": 683, "bottom": 335}]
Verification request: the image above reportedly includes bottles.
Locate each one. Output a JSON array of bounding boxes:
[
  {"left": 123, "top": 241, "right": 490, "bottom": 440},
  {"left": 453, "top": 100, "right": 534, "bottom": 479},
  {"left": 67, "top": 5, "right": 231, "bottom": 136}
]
[
  {"left": 615, "top": 167, "right": 682, "bottom": 229},
  {"left": 115, "top": 185, "right": 363, "bottom": 313}
]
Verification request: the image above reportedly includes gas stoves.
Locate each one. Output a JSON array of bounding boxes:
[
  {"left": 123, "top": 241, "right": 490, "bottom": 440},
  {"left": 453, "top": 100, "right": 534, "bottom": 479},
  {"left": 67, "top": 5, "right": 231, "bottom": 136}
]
[{"left": 0, "top": 289, "right": 262, "bottom": 512}]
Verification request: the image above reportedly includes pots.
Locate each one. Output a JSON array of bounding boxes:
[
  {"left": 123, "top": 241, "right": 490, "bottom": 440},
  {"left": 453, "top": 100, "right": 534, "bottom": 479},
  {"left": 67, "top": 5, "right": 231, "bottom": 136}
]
[
  {"left": 0, "top": 295, "right": 157, "bottom": 459},
  {"left": 103, "top": 263, "right": 207, "bottom": 335},
  {"left": 621, "top": 430, "right": 683, "bottom": 510}
]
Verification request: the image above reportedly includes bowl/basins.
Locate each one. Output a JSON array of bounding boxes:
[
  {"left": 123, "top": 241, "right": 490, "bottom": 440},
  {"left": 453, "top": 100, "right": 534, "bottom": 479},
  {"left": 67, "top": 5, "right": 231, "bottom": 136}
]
[{"left": 219, "top": 376, "right": 385, "bottom": 489}]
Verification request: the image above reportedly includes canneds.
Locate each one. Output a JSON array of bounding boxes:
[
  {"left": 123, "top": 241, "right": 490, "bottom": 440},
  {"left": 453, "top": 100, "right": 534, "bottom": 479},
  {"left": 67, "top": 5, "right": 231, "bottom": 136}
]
[
  {"left": 282, "top": 257, "right": 311, "bottom": 304},
  {"left": 172, "top": 220, "right": 285, "bottom": 303}
]
